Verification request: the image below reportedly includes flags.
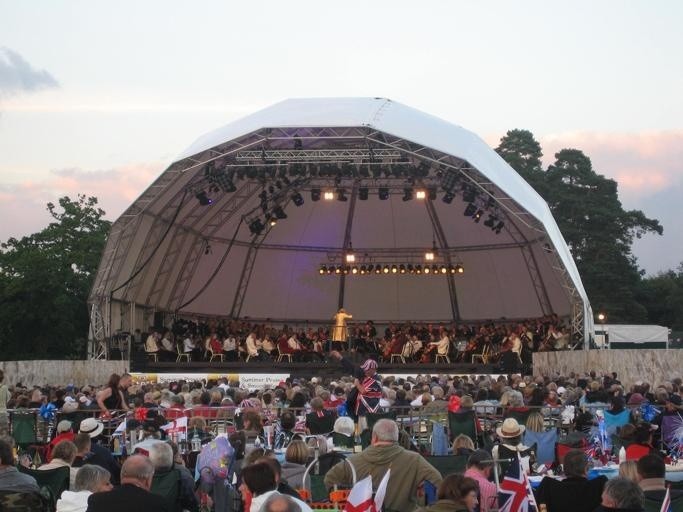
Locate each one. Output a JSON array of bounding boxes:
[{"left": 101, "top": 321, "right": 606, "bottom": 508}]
[
  {"left": 659, "top": 485, "right": 672, "bottom": 512},
  {"left": 497, "top": 450, "right": 538, "bottom": 512}
]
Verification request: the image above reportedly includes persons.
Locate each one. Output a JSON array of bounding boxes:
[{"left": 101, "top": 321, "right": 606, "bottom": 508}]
[
  {"left": 85, "top": 453, "right": 170, "bottom": 512},
  {"left": 55, "top": 464, "right": 114, "bottom": 512},
  {"left": 8, "top": 368, "right": 99, "bottom": 410},
  {"left": 323, "top": 418, "right": 443, "bottom": 512},
  {"left": 233, "top": 351, "right": 380, "bottom": 452},
  {"left": 595, "top": 477, "right": 647, "bottom": 511},
  {"left": 43, "top": 419, "right": 77, "bottom": 463},
  {"left": 119, "top": 373, "right": 132, "bottom": 415},
  {"left": 380, "top": 368, "right": 503, "bottom": 455},
  {"left": 464, "top": 449, "right": 501, "bottom": 512},
  {"left": 37, "top": 438, "right": 81, "bottom": 490},
  {"left": 332, "top": 308, "right": 352, "bottom": 352},
  {"left": 503, "top": 368, "right": 559, "bottom": 432},
  {"left": 0, "top": 370, "right": 12, "bottom": 438},
  {"left": 96, "top": 373, "right": 133, "bottom": 421},
  {"left": 133, "top": 312, "right": 328, "bottom": 362},
  {"left": 128, "top": 378, "right": 233, "bottom": 448},
  {"left": 201, "top": 431, "right": 247, "bottom": 512},
  {"left": 255, "top": 456, "right": 301, "bottom": 499},
  {"left": 238, "top": 463, "right": 313, "bottom": 512},
  {"left": 257, "top": 494, "right": 302, "bottom": 512},
  {"left": 559, "top": 368, "right": 683, "bottom": 464},
  {"left": 149, "top": 441, "right": 182, "bottom": 511},
  {"left": 71, "top": 433, "right": 92, "bottom": 467},
  {"left": 636, "top": 454, "right": 683, "bottom": 512},
  {"left": 492, "top": 417, "right": 538, "bottom": 489},
  {"left": 167, "top": 441, "right": 196, "bottom": 512},
  {"left": 618, "top": 461, "right": 641, "bottom": 484},
  {"left": 354, "top": 321, "right": 376, "bottom": 351},
  {"left": 414, "top": 473, "right": 480, "bottom": 512},
  {"left": 77, "top": 418, "right": 122, "bottom": 482},
  {"left": 535, "top": 450, "right": 606, "bottom": 512},
  {"left": 281, "top": 439, "right": 312, "bottom": 501},
  {"left": 0, "top": 436, "right": 41, "bottom": 497},
  {"left": 378, "top": 314, "right": 564, "bottom": 363}
]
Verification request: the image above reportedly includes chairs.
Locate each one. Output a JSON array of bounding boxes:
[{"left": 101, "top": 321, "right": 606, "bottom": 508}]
[
  {"left": 435, "top": 344, "right": 449, "bottom": 363},
  {"left": 471, "top": 344, "right": 489, "bottom": 365},
  {"left": 143, "top": 343, "right": 159, "bottom": 362},
  {"left": 390, "top": 344, "right": 405, "bottom": 364},
  {"left": 176, "top": 343, "right": 192, "bottom": 363},
  {"left": 210, "top": 344, "right": 225, "bottom": 362},
  {"left": 277, "top": 344, "right": 293, "bottom": 363}
]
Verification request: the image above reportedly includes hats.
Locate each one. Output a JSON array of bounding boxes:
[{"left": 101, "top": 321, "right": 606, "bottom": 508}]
[
  {"left": 627, "top": 393, "right": 649, "bottom": 404},
  {"left": 496, "top": 418, "right": 526, "bottom": 439},
  {"left": 62, "top": 402, "right": 79, "bottom": 413},
  {"left": 557, "top": 386, "right": 566, "bottom": 395},
  {"left": 64, "top": 396, "right": 75, "bottom": 402},
  {"left": 360, "top": 359, "right": 378, "bottom": 371},
  {"left": 57, "top": 419, "right": 74, "bottom": 433},
  {"left": 78, "top": 417, "right": 104, "bottom": 437},
  {"left": 79, "top": 396, "right": 92, "bottom": 406},
  {"left": 239, "top": 399, "right": 256, "bottom": 407},
  {"left": 152, "top": 391, "right": 165, "bottom": 400},
  {"left": 334, "top": 387, "right": 345, "bottom": 397},
  {"left": 664, "top": 395, "right": 683, "bottom": 406}
]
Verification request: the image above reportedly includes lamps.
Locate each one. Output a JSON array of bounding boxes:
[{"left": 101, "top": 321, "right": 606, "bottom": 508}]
[{"left": 320, "top": 250, "right": 464, "bottom": 274}]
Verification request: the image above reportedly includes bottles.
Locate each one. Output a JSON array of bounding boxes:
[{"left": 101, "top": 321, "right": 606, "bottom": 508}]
[
  {"left": 529, "top": 450, "right": 538, "bottom": 476},
  {"left": 619, "top": 446, "right": 627, "bottom": 465},
  {"left": 113, "top": 436, "right": 119, "bottom": 453},
  {"left": 138, "top": 424, "right": 145, "bottom": 443},
  {"left": 353, "top": 423, "right": 363, "bottom": 453},
  {"left": 255, "top": 435, "right": 261, "bottom": 448},
  {"left": 181, "top": 433, "right": 187, "bottom": 452},
  {"left": 173, "top": 432, "right": 178, "bottom": 447},
  {"left": 263, "top": 426, "right": 275, "bottom": 450},
  {"left": 191, "top": 424, "right": 202, "bottom": 451}
]
